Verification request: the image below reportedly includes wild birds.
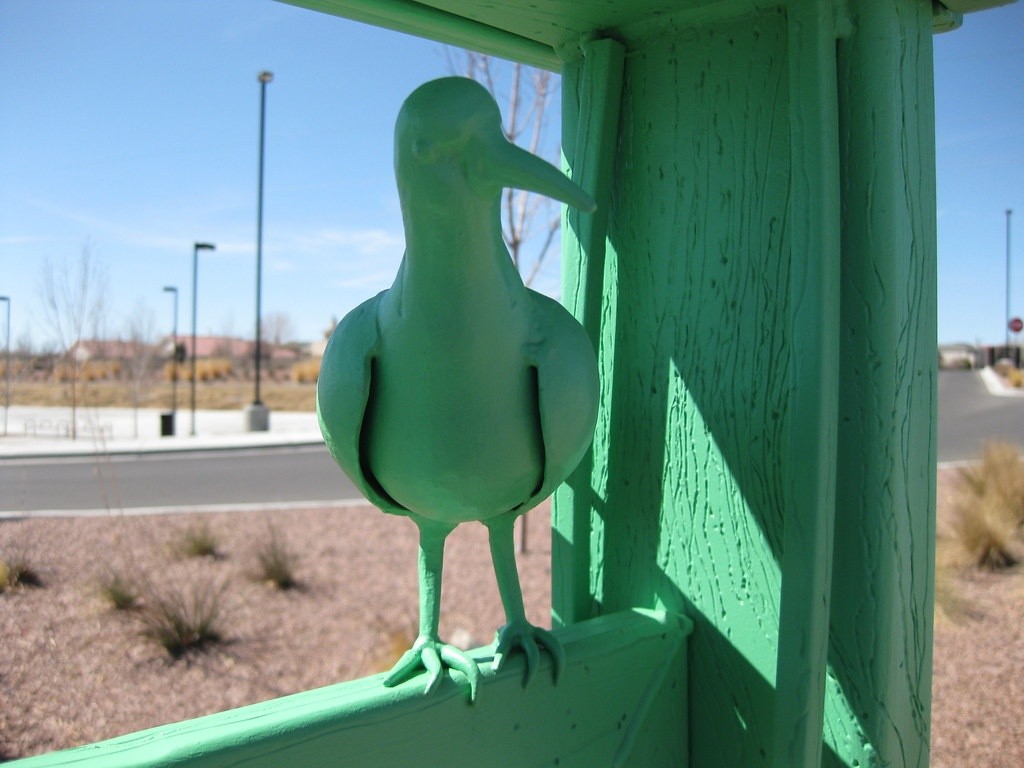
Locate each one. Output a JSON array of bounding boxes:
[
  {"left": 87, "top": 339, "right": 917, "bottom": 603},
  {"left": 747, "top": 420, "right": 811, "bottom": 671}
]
[{"left": 313, "top": 77, "right": 602, "bottom": 705}]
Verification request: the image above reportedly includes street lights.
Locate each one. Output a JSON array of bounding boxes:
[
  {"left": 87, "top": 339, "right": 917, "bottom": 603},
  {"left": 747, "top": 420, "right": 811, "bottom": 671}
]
[
  {"left": 190, "top": 244, "right": 215, "bottom": 437},
  {"left": 1005, "top": 210, "right": 1012, "bottom": 345},
  {"left": 164, "top": 286, "right": 178, "bottom": 435},
  {"left": 0, "top": 296, "right": 10, "bottom": 435},
  {"left": 245, "top": 71, "right": 273, "bottom": 432}
]
